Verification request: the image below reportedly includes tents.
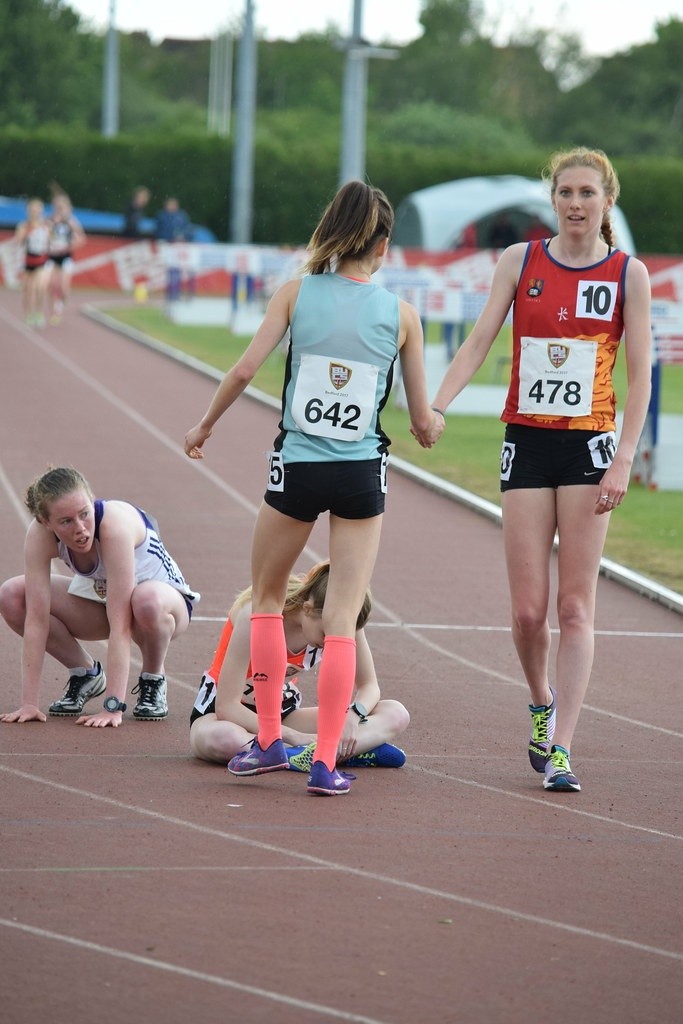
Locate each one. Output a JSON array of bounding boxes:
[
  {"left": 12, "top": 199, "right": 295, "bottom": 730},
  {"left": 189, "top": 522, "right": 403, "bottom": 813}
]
[{"left": 392, "top": 173, "right": 639, "bottom": 260}]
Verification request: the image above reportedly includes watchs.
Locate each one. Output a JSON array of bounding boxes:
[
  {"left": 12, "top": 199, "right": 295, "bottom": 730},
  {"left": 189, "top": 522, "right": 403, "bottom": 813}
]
[
  {"left": 103, "top": 696, "right": 126, "bottom": 712},
  {"left": 350, "top": 702, "right": 368, "bottom": 722}
]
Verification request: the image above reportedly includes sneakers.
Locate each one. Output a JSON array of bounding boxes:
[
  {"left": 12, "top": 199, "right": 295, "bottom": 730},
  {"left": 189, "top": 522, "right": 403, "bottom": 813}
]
[
  {"left": 227, "top": 735, "right": 290, "bottom": 776},
  {"left": 285, "top": 742, "right": 317, "bottom": 773},
  {"left": 338, "top": 743, "right": 406, "bottom": 767},
  {"left": 542, "top": 744, "right": 580, "bottom": 791},
  {"left": 49, "top": 660, "right": 107, "bottom": 717},
  {"left": 130, "top": 671, "right": 168, "bottom": 721},
  {"left": 25, "top": 312, "right": 62, "bottom": 327},
  {"left": 306, "top": 759, "right": 351, "bottom": 795},
  {"left": 528, "top": 683, "right": 557, "bottom": 774}
]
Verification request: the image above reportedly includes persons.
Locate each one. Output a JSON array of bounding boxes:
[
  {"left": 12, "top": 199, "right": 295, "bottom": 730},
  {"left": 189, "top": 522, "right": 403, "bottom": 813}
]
[
  {"left": 413, "top": 148, "right": 652, "bottom": 788},
  {"left": 0, "top": 466, "right": 200, "bottom": 727},
  {"left": 14, "top": 196, "right": 82, "bottom": 329},
  {"left": 125, "top": 186, "right": 192, "bottom": 240},
  {"left": 185, "top": 183, "right": 446, "bottom": 796},
  {"left": 189, "top": 559, "right": 411, "bottom": 771}
]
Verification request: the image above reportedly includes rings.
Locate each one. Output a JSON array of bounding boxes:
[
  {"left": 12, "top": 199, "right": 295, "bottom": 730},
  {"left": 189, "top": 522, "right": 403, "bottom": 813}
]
[
  {"left": 601, "top": 496, "right": 608, "bottom": 501},
  {"left": 343, "top": 748, "right": 347, "bottom": 749},
  {"left": 608, "top": 500, "right": 613, "bottom": 503}
]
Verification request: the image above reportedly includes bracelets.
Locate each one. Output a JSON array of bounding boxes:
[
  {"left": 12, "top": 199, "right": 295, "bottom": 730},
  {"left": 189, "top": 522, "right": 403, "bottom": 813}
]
[{"left": 432, "top": 407, "right": 444, "bottom": 417}]
